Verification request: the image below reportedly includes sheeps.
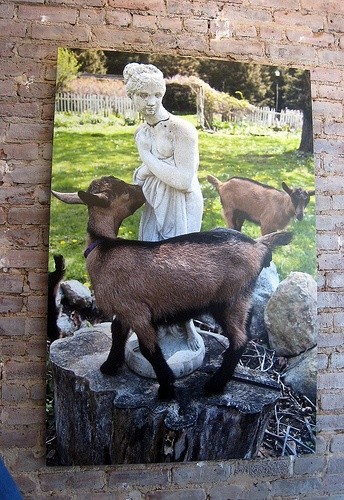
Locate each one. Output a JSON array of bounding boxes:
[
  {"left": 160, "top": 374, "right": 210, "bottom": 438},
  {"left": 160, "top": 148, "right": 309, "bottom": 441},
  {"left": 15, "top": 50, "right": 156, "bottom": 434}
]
[
  {"left": 51, "top": 176, "right": 293, "bottom": 401},
  {"left": 206, "top": 176, "right": 315, "bottom": 236}
]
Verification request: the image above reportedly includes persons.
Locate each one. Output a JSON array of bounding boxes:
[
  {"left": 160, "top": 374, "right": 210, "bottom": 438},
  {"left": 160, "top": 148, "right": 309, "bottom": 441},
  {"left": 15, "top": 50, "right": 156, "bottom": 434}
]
[{"left": 120, "top": 63, "right": 205, "bottom": 378}]
